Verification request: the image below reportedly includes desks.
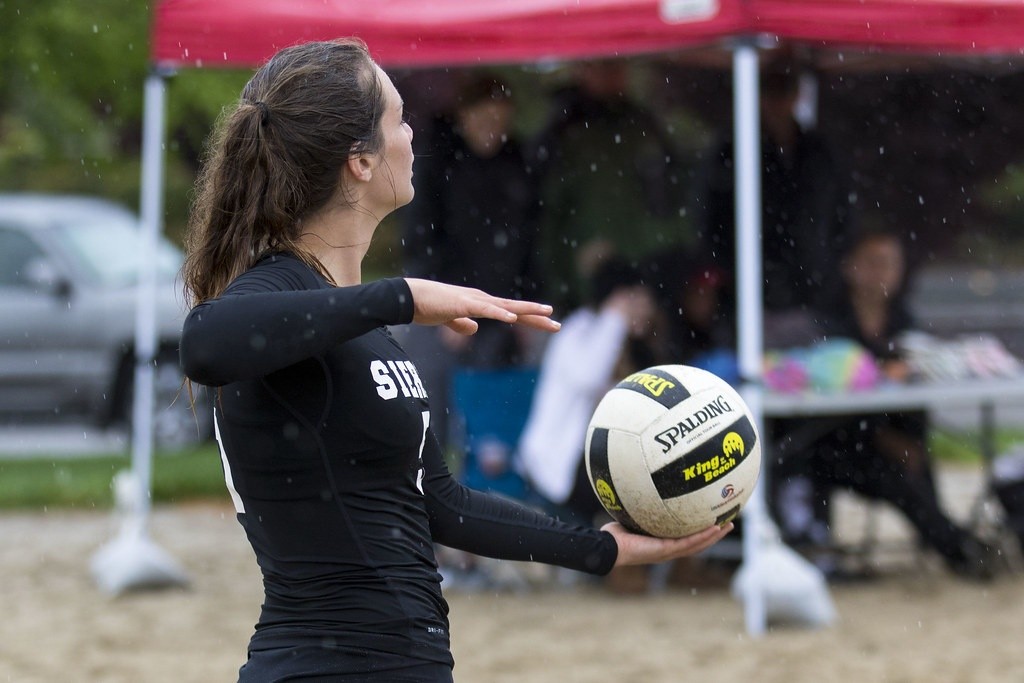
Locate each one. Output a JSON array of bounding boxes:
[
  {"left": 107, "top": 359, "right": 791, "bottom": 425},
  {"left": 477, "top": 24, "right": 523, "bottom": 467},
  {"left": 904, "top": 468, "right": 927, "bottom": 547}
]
[{"left": 764, "top": 376, "right": 1024, "bottom": 577}]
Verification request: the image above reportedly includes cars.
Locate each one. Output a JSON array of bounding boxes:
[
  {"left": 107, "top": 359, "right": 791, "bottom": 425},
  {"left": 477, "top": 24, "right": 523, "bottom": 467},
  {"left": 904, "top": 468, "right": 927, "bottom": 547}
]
[{"left": 0, "top": 193, "right": 216, "bottom": 454}]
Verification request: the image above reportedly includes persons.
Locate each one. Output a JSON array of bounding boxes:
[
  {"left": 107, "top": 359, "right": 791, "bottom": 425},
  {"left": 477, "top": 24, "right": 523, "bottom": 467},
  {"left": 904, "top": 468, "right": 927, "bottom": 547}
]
[
  {"left": 175, "top": 37, "right": 734, "bottom": 683},
  {"left": 353, "top": 35, "right": 1024, "bottom": 588}
]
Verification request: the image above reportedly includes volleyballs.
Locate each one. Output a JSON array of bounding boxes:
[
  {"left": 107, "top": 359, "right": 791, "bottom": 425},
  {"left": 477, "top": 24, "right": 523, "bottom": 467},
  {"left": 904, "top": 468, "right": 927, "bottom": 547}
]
[{"left": 583, "top": 363, "right": 761, "bottom": 537}]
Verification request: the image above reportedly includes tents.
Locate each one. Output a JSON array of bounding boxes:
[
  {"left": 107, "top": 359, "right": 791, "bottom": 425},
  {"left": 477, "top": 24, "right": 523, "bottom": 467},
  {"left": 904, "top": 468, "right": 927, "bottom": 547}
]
[{"left": 88, "top": 0, "right": 1024, "bottom": 639}]
